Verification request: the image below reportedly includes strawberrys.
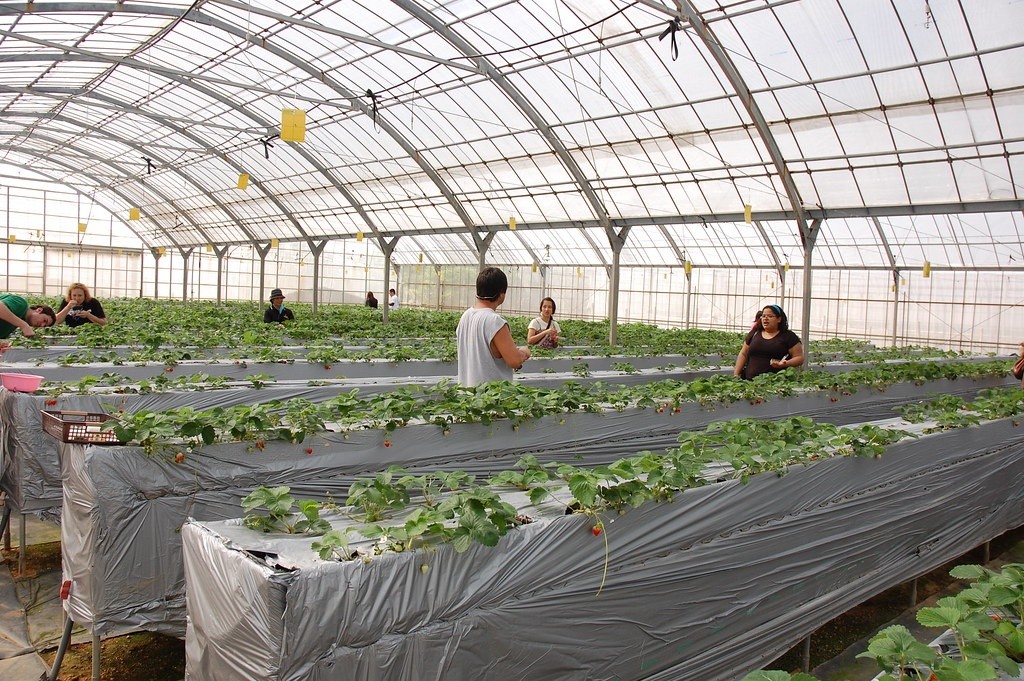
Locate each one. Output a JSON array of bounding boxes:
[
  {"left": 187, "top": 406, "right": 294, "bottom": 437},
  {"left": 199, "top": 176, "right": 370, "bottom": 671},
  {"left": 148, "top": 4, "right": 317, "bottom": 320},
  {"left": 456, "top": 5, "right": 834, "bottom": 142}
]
[
  {"left": 384, "top": 441, "right": 390, "bottom": 447},
  {"left": 256, "top": 441, "right": 265, "bottom": 447},
  {"left": 660, "top": 408, "right": 663, "bottom": 413},
  {"left": 675, "top": 408, "right": 680, "bottom": 413},
  {"left": 176, "top": 452, "right": 184, "bottom": 462},
  {"left": 592, "top": 526, "right": 601, "bottom": 536},
  {"left": 751, "top": 399, "right": 762, "bottom": 405},
  {"left": 306, "top": 448, "right": 312, "bottom": 454}
]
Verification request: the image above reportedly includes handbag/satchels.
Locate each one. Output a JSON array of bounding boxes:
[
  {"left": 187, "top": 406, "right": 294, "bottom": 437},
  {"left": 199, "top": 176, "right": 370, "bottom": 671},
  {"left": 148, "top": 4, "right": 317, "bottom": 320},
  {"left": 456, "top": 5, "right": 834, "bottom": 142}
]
[{"left": 532, "top": 329, "right": 553, "bottom": 349}]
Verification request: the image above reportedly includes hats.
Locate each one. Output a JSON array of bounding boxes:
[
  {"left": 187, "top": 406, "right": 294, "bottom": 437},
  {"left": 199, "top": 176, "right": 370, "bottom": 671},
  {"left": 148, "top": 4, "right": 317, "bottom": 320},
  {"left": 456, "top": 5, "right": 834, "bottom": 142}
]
[{"left": 270, "top": 289, "right": 286, "bottom": 300}]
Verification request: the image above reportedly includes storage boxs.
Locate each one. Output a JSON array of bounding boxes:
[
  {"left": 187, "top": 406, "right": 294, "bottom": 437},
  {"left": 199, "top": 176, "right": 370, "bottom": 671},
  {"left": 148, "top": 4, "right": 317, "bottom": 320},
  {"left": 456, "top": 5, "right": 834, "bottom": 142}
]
[{"left": 40, "top": 408, "right": 126, "bottom": 447}]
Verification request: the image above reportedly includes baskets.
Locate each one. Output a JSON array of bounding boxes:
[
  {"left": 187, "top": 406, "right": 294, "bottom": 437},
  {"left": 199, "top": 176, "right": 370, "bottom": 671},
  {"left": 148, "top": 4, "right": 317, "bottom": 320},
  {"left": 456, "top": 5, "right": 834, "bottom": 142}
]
[{"left": 40, "top": 408, "right": 129, "bottom": 446}]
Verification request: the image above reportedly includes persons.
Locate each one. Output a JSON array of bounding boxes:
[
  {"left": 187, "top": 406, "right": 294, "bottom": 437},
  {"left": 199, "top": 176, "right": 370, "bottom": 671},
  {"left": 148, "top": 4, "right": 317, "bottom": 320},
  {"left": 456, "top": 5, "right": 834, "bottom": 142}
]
[
  {"left": 456, "top": 267, "right": 531, "bottom": 388},
  {"left": 734, "top": 306, "right": 804, "bottom": 381},
  {"left": 56, "top": 283, "right": 108, "bottom": 327},
  {"left": 0, "top": 293, "right": 56, "bottom": 339},
  {"left": 527, "top": 297, "right": 561, "bottom": 348},
  {"left": 388, "top": 289, "right": 400, "bottom": 309},
  {"left": 365, "top": 292, "right": 377, "bottom": 308},
  {"left": 264, "top": 288, "right": 294, "bottom": 324}
]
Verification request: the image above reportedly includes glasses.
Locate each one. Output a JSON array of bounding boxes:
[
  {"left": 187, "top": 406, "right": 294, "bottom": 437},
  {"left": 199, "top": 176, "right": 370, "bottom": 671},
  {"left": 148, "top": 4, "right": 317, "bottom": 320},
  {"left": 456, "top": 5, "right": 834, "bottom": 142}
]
[
  {"left": 475, "top": 295, "right": 497, "bottom": 300},
  {"left": 760, "top": 315, "right": 778, "bottom": 319}
]
